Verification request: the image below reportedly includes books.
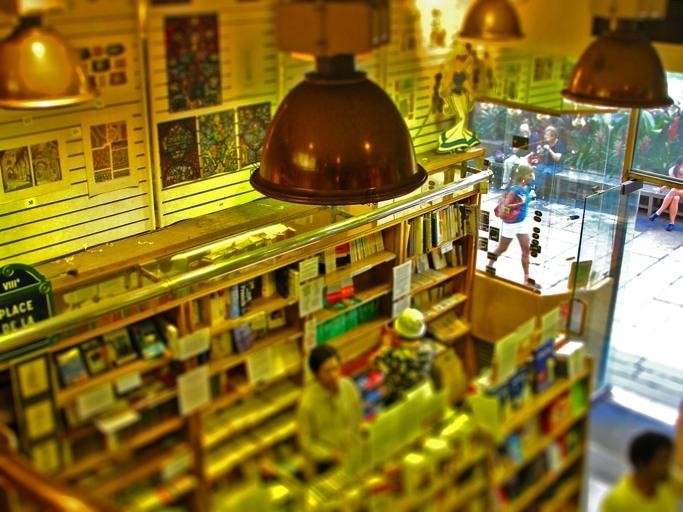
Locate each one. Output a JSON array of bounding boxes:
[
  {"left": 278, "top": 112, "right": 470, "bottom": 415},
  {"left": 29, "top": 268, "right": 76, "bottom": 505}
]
[
  {"left": 299, "top": 370, "right": 490, "bottom": 512},
  {"left": 56, "top": 224, "right": 299, "bottom": 512},
  {"left": 490, "top": 304, "right": 587, "bottom": 511},
  {"left": 299, "top": 201, "right": 477, "bottom": 350}
]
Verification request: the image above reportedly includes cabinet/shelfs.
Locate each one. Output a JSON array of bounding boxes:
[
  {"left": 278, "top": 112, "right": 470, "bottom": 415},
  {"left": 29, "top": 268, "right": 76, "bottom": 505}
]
[{"left": 0, "top": 177, "right": 592, "bottom": 511}]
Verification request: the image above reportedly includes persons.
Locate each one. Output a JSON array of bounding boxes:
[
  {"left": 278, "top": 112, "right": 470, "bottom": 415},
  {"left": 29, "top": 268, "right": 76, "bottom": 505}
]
[
  {"left": 295, "top": 343, "right": 364, "bottom": 475},
  {"left": 649, "top": 155, "right": 683, "bottom": 231},
  {"left": 367, "top": 308, "right": 435, "bottom": 407},
  {"left": 599, "top": 433, "right": 683, "bottom": 512},
  {"left": 500, "top": 123, "right": 563, "bottom": 204},
  {"left": 486, "top": 164, "right": 542, "bottom": 289}
]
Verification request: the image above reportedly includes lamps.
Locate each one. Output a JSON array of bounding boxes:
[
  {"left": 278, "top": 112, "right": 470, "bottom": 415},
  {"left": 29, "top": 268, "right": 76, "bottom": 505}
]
[
  {"left": 560, "top": 0, "right": 675, "bottom": 107},
  {"left": 0, "top": 0, "right": 92, "bottom": 109},
  {"left": 251, "top": 0, "right": 427, "bottom": 201},
  {"left": 457, "top": 0, "right": 523, "bottom": 42}
]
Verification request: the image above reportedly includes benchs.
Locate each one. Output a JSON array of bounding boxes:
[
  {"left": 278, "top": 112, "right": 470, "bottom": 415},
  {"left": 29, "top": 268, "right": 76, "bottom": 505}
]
[{"left": 478, "top": 153, "right": 682, "bottom": 215}]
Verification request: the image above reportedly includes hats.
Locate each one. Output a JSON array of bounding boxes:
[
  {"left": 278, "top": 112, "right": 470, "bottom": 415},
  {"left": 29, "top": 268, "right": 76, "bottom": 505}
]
[
  {"left": 393, "top": 309, "right": 427, "bottom": 340},
  {"left": 514, "top": 163, "right": 537, "bottom": 183}
]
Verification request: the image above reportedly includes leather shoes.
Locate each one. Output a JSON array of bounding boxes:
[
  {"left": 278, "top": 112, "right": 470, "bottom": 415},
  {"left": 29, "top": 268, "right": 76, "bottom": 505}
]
[
  {"left": 648, "top": 213, "right": 659, "bottom": 222},
  {"left": 665, "top": 224, "right": 675, "bottom": 231}
]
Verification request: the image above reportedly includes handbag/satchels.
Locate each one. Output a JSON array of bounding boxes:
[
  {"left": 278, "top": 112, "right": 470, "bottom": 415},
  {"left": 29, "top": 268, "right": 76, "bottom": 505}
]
[{"left": 494, "top": 198, "right": 520, "bottom": 223}]
